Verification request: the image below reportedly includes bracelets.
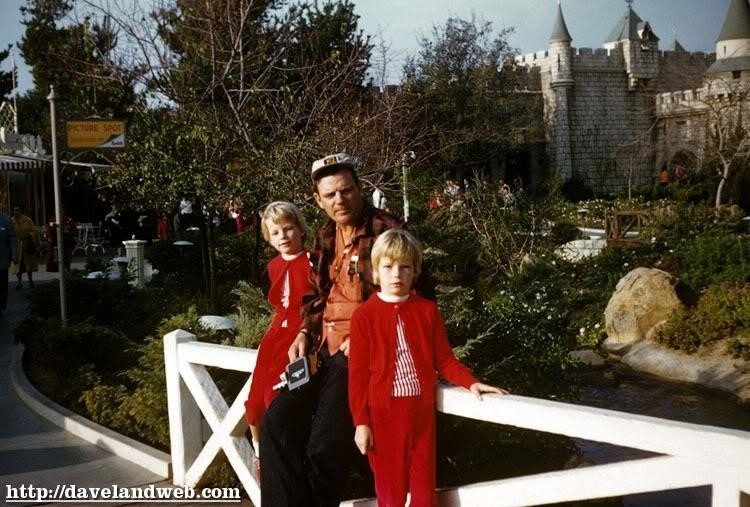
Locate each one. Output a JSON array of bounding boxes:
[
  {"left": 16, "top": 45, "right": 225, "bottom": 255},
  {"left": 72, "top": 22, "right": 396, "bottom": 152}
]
[{"left": 296, "top": 328, "right": 309, "bottom": 337}]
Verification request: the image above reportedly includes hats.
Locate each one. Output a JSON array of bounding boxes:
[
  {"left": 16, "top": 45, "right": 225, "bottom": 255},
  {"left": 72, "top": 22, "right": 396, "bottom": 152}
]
[{"left": 312, "top": 153, "right": 356, "bottom": 181}]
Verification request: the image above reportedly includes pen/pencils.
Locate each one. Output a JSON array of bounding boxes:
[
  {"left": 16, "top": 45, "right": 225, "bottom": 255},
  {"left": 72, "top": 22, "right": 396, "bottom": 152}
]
[{"left": 354, "top": 261, "right": 358, "bottom": 273}]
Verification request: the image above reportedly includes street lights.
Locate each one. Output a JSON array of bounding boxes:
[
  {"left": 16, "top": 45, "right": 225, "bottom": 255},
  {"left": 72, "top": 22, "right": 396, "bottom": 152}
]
[{"left": 401, "top": 150, "right": 416, "bottom": 221}]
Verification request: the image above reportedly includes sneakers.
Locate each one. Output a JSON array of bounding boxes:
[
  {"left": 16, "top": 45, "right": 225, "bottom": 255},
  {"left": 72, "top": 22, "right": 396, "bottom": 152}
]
[{"left": 254, "top": 454, "right": 260, "bottom": 485}]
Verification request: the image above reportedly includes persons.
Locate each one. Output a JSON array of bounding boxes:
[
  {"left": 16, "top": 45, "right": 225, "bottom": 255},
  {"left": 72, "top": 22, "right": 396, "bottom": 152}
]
[
  {"left": 372, "top": 177, "right": 387, "bottom": 211},
  {"left": 659, "top": 163, "right": 669, "bottom": 188},
  {"left": 258, "top": 152, "right": 435, "bottom": 506},
  {"left": 0, "top": 199, "right": 244, "bottom": 311},
  {"left": 245, "top": 201, "right": 312, "bottom": 484},
  {"left": 429, "top": 177, "right": 524, "bottom": 213},
  {"left": 349, "top": 228, "right": 509, "bottom": 506}
]
